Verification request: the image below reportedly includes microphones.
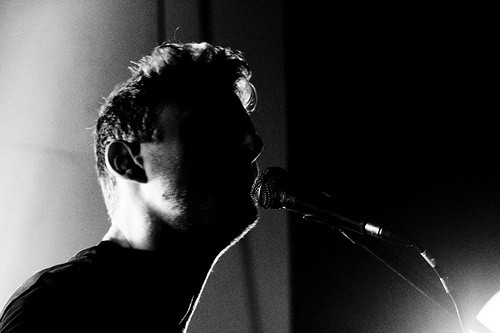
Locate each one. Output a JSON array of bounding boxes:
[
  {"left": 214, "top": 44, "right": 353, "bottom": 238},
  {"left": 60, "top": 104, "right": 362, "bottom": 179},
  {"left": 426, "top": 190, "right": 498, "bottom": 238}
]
[{"left": 250, "top": 164, "right": 415, "bottom": 248}]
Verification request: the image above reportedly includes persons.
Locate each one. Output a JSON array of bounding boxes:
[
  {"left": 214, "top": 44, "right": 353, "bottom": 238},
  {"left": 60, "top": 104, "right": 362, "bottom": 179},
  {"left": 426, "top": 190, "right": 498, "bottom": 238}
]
[{"left": 0, "top": 26, "right": 265, "bottom": 333}]
